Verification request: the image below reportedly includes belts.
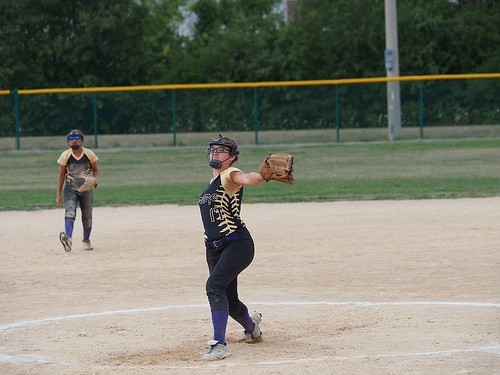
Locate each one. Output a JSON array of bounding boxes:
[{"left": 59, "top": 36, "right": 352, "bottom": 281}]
[{"left": 204, "top": 231, "right": 237, "bottom": 250}]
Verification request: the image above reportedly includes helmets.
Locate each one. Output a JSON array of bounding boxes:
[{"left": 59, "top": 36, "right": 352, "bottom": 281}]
[
  {"left": 205, "top": 134, "right": 239, "bottom": 169},
  {"left": 66, "top": 129, "right": 85, "bottom": 150}
]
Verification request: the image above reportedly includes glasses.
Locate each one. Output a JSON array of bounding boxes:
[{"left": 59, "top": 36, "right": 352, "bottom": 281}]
[
  {"left": 68, "top": 135, "right": 80, "bottom": 140},
  {"left": 207, "top": 147, "right": 230, "bottom": 154}
]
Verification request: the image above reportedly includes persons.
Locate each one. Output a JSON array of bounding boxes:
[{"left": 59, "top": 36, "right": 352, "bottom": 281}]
[
  {"left": 56, "top": 128, "right": 100, "bottom": 253},
  {"left": 198, "top": 136, "right": 265, "bottom": 361}
]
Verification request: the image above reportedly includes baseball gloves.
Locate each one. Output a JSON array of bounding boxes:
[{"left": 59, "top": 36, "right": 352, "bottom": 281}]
[
  {"left": 259, "top": 153, "right": 295, "bottom": 185},
  {"left": 73, "top": 177, "right": 98, "bottom": 192}
]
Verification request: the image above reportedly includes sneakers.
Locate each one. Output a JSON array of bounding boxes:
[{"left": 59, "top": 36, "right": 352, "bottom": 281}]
[
  {"left": 203, "top": 340, "right": 231, "bottom": 361},
  {"left": 243, "top": 311, "right": 262, "bottom": 343},
  {"left": 59, "top": 232, "right": 72, "bottom": 252},
  {"left": 82, "top": 240, "right": 94, "bottom": 250}
]
